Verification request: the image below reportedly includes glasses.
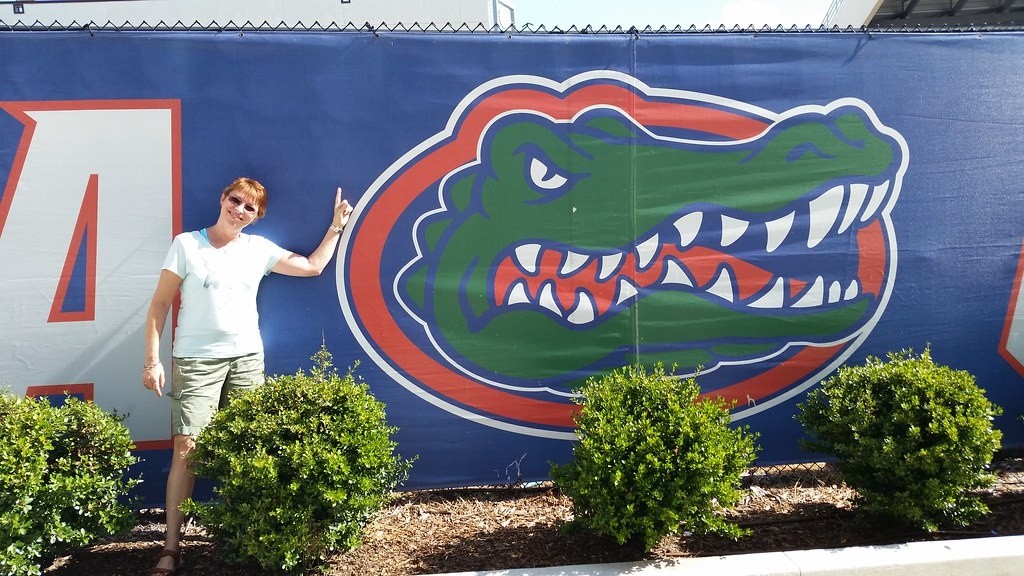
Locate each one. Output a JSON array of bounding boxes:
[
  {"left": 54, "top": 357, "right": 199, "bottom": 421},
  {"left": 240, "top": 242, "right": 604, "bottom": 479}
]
[{"left": 226, "top": 194, "right": 259, "bottom": 214}]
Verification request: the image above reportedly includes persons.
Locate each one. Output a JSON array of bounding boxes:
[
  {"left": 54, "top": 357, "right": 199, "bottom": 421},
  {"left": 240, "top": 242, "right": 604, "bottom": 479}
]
[{"left": 138, "top": 177, "right": 353, "bottom": 576}]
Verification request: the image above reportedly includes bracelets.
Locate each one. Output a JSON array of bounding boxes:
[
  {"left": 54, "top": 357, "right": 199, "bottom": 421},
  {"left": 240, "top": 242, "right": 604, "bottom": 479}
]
[
  {"left": 329, "top": 224, "right": 344, "bottom": 234},
  {"left": 143, "top": 360, "right": 161, "bottom": 375}
]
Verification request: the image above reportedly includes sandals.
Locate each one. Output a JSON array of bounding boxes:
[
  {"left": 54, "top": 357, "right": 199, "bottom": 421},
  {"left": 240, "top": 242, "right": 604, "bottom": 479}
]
[{"left": 150, "top": 549, "right": 185, "bottom": 576}]
